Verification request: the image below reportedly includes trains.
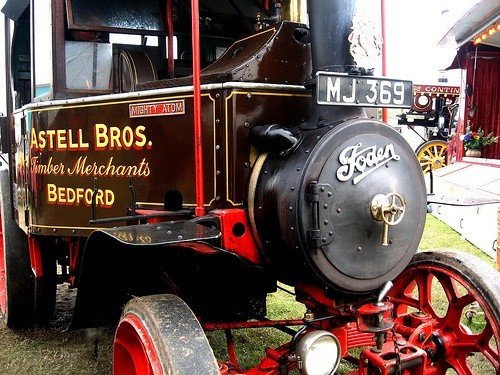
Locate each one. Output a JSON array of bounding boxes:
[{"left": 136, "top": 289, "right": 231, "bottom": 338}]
[{"left": 0, "top": 0, "right": 500, "bottom": 374}]
